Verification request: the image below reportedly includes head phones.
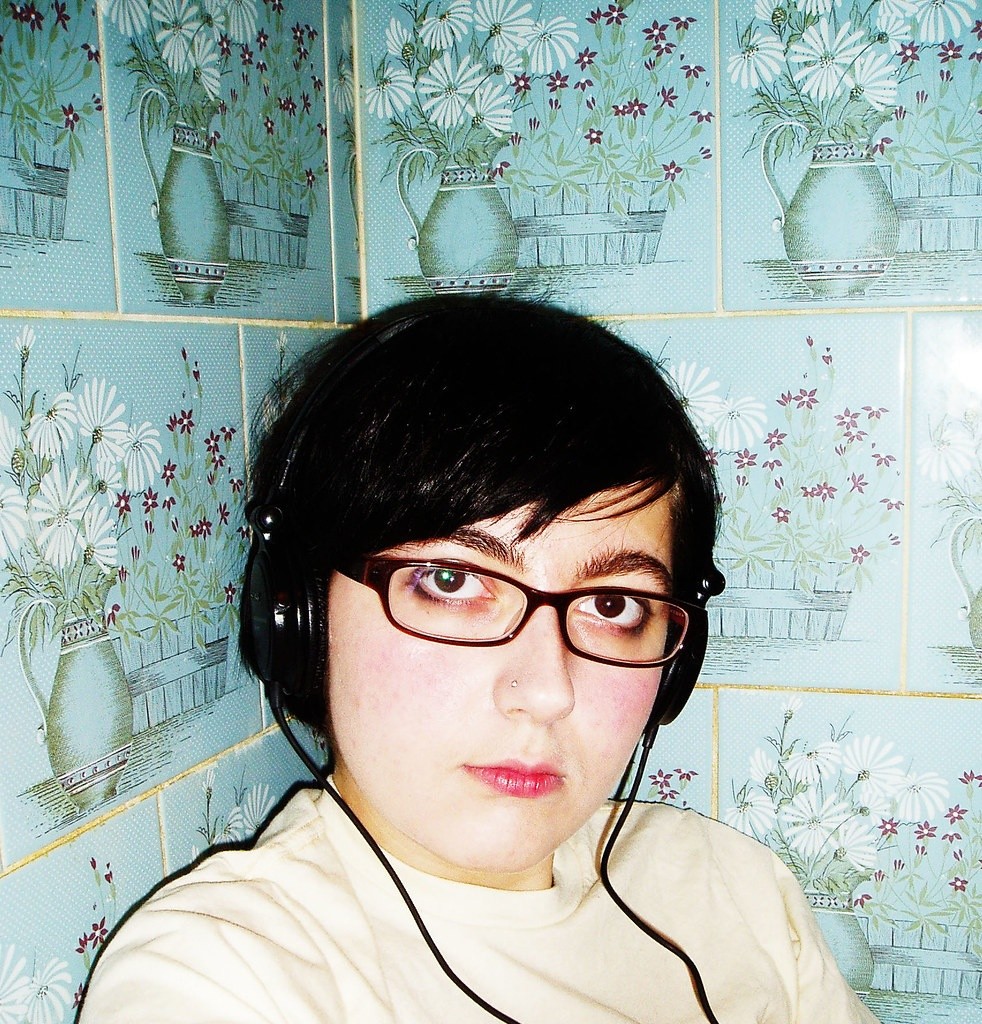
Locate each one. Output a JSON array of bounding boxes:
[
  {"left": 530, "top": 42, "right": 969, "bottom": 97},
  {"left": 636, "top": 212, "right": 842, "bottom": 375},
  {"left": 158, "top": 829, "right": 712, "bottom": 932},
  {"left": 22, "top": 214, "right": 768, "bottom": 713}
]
[{"left": 238, "top": 291, "right": 729, "bottom": 750}]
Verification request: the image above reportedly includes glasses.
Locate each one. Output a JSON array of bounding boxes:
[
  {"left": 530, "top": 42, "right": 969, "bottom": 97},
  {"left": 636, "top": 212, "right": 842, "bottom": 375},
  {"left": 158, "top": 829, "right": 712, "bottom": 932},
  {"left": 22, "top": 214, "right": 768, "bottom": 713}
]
[{"left": 337, "top": 550, "right": 708, "bottom": 669}]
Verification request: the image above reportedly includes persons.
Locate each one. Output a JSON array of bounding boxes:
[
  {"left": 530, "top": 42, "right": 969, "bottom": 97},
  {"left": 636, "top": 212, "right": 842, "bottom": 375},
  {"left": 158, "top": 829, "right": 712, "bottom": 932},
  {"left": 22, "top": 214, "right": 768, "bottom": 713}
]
[{"left": 70, "top": 293, "right": 882, "bottom": 1024}]
